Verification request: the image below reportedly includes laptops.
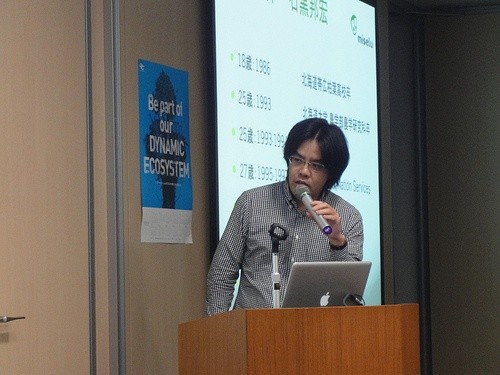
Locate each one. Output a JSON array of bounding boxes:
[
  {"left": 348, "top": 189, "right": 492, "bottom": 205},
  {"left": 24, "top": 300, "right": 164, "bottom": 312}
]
[{"left": 281, "top": 262, "right": 372, "bottom": 308}]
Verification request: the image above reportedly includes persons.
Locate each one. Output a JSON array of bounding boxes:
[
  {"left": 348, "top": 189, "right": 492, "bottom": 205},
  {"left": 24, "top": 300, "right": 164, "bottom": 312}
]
[{"left": 204, "top": 117, "right": 366, "bottom": 320}]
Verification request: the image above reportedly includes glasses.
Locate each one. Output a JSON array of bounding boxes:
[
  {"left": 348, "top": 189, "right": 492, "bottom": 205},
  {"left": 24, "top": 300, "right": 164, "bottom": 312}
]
[{"left": 287, "top": 153, "right": 330, "bottom": 174}]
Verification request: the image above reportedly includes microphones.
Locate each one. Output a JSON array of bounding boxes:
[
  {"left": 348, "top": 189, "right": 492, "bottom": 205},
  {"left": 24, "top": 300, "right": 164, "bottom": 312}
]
[{"left": 296, "top": 184, "right": 332, "bottom": 235}]
